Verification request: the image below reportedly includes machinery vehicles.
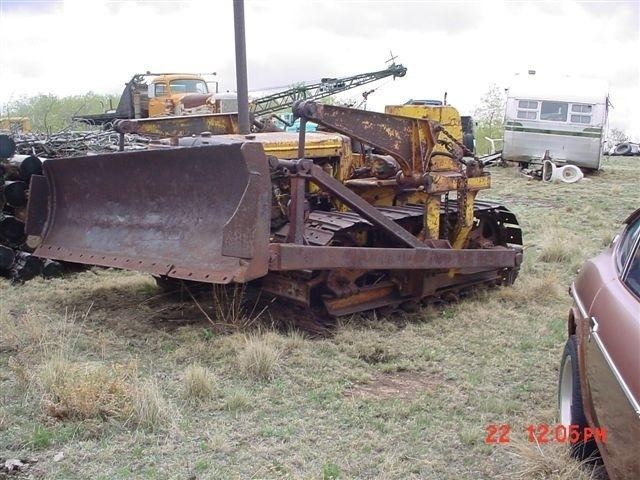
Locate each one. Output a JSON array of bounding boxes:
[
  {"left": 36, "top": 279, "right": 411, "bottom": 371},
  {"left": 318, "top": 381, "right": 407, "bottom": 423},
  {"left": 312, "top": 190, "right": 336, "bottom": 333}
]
[{"left": 23, "top": 49, "right": 524, "bottom": 337}]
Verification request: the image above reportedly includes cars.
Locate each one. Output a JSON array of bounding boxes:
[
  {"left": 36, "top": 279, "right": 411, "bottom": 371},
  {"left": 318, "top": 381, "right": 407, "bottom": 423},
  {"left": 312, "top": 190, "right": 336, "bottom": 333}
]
[{"left": 555, "top": 207, "right": 640, "bottom": 480}]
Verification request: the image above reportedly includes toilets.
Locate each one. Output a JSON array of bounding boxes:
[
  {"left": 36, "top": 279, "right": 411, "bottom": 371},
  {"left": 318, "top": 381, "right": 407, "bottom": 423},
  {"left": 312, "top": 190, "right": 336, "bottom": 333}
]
[{"left": 542, "top": 160, "right": 584, "bottom": 184}]
